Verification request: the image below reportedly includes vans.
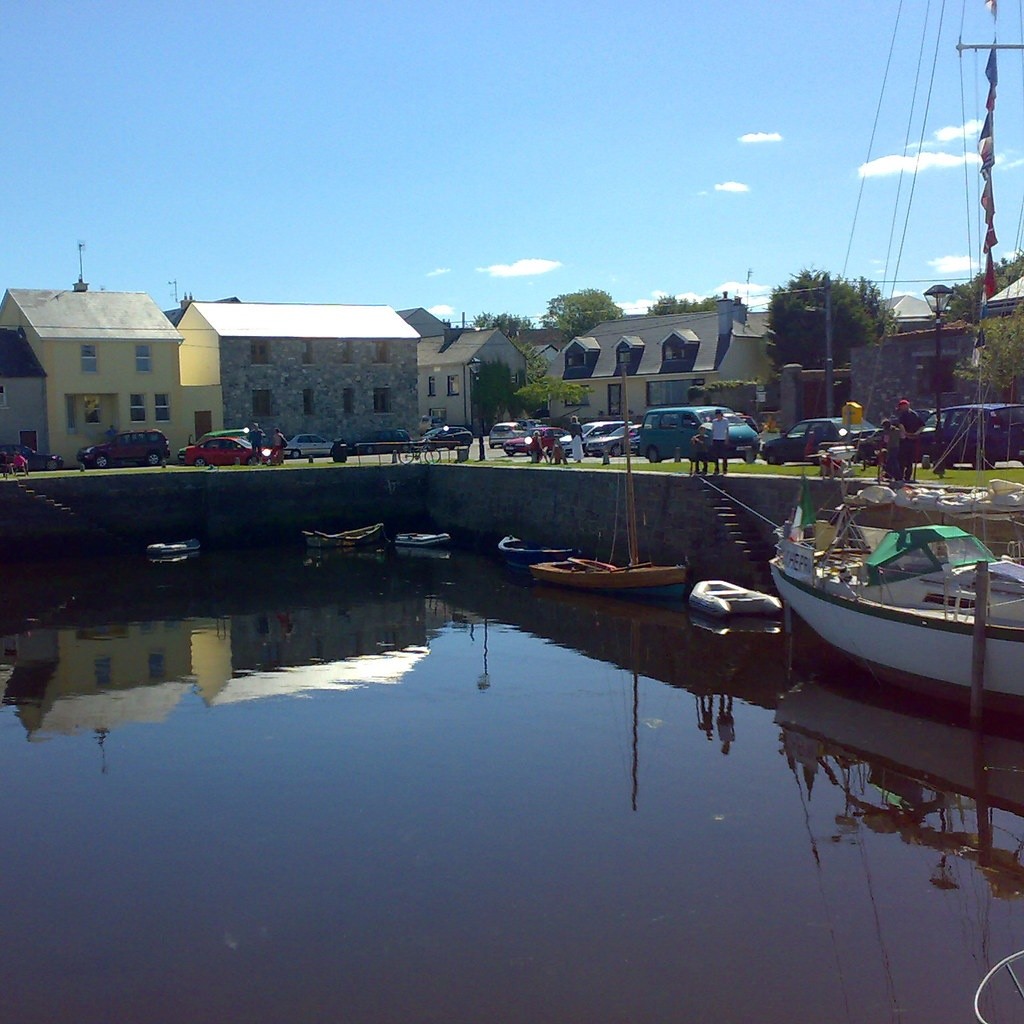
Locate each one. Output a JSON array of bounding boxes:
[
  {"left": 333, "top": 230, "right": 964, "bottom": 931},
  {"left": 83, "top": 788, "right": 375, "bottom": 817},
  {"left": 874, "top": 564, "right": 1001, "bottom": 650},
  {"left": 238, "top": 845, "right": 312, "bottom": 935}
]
[{"left": 638, "top": 405, "right": 762, "bottom": 464}]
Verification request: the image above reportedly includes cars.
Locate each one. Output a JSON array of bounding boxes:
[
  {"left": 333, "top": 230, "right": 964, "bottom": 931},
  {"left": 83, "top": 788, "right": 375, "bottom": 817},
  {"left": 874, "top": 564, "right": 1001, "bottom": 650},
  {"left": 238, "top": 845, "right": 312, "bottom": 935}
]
[
  {"left": 1, "top": 424, "right": 280, "bottom": 468},
  {"left": 419, "top": 414, "right": 447, "bottom": 434},
  {"left": 489, "top": 418, "right": 641, "bottom": 458},
  {"left": 347, "top": 428, "right": 413, "bottom": 454},
  {"left": 284, "top": 433, "right": 335, "bottom": 458},
  {"left": 413, "top": 426, "right": 473, "bottom": 453},
  {"left": 763, "top": 403, "right": 1023, "bottom": 472}
]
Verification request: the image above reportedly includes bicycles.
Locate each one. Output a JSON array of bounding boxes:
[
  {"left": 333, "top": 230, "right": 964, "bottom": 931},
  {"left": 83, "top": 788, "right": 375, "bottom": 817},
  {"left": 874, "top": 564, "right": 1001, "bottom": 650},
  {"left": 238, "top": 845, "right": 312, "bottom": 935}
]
[{"left": 399, "top": 439, "right": 442, "bottom": 465}]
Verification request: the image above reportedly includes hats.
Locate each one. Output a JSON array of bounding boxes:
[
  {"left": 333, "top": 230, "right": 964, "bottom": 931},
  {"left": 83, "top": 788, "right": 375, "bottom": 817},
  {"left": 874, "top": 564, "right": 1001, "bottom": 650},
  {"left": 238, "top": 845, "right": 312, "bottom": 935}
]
[
  {"left": 898, "top": 400, "right": 910, "bottom": 406},
  {"left": 881, "top": 420, "right": 891, "bottom": 427}
]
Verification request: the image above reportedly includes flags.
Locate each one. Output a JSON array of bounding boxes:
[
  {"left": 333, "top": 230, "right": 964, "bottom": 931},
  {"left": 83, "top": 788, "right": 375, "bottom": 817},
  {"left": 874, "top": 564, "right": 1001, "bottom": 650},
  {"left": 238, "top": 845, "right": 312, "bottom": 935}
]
[
  {"left": 971, "top": 0, "right": 997, "bottom": 368},
  {"left": 799, "top": 468, "right": 816, "bottom": 524}
]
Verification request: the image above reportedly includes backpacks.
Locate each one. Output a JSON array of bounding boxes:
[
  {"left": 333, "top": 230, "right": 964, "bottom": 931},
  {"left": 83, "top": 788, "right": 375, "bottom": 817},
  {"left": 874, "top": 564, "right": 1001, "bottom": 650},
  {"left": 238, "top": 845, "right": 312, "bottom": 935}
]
[{"left": 531, "top": 437, "right": 539, "bottom": 448}]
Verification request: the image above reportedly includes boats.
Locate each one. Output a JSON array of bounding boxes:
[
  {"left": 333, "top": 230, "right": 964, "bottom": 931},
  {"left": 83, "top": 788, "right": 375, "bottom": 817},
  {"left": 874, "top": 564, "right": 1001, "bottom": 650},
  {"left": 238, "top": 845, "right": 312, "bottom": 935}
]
[
  {"left": 147, "top": 538, "right": 202, "bottom": 553},
  {"left": 301, "top": 548, "right": 454, "bottom": 572},
  {"left": 148, "top": 555, "right": 197, "bottom": 563},
  {"left": 496, "top": 535, "right": 584, "bottom": 569},
  {"left": 686, "top": 578, "right": 782, "bottom": 622},
  {"left": 303, "top": 523, "right": 451, "bottom": 548}
]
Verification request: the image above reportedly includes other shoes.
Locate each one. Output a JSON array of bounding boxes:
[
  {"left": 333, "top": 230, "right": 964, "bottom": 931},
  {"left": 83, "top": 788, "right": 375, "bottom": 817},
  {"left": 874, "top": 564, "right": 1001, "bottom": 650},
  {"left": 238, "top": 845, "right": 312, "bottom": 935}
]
[
  {"left": 904, "top": 476, "right": 911, "bottom": 481},
  {"left": 882, "top": 474, "right": 892, "bottom": 481},
  {"left": 713, "top": 472, "right": 719, "bottom": 475},
  {"left": 894, "top": 477, "right": 904, "bottom": 483}
]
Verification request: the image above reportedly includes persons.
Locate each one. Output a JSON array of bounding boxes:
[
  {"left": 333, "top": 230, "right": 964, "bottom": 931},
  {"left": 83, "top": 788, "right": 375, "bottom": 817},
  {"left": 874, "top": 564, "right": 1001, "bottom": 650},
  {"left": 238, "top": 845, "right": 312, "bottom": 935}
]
[
  {"left": 0, "top": 451, "right": 12, "bottom": 474},
  {"left": 568, "top": 417, "right": 584, "bottom": 463},
  {"left": 270, "top": 428, "right": 284, "bottom": 466},
  {"left": 711, "top": 411, "right": 730, "bottom": 475},
  {"left": 275, "top": 609, "right": 293, "bottom": 635},
  {"left": 691, "top": 426, "right": 709, "bottom": 474},
  {"left": 698, "top": 694, "right": 714, "bottom": 740},
  {"left": 86, "top": 401, "right": 98, "bottom": 422},
  {"left": 880, "top": 399, "right": 926, "bottom": 482},
  {"left": 419, "top": 416, "right": 430, "bottom": 436},
  {"left": 13, "top": 450, "right": 29, "bottom": 476},
  {"left": 531, "top": 430, "right": 569, "bottom": 464},
  {"left": 716, "top": 694, "right": 735, "bottom": 755},
  {"left": 105, "top": 425, "right": 119, "bottom": 441},
  {"left": 248, "top": 423, "right": 264, "bottom": 464}
]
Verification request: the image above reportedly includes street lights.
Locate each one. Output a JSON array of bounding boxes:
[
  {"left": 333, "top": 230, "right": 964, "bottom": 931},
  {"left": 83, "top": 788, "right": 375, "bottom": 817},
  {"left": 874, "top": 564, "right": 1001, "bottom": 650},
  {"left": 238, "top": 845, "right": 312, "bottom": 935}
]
[{"left": 922, "top": 286, "right": 956, "bottom": 471}]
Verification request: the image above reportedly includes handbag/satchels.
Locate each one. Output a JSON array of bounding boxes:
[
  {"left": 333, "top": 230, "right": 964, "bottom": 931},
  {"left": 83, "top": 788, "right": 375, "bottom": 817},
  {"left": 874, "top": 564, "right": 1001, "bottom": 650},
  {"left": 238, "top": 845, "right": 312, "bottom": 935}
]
[{"left": 281, "top": 437, "right": 288, "bottom": 448}]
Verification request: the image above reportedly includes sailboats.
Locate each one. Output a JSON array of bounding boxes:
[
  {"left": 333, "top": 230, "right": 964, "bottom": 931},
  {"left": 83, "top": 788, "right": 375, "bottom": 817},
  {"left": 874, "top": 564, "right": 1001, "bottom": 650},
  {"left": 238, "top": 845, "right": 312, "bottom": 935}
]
[
  {"left": 768, "top": 655, "right": 1023, "bottom": 1023},
  {"left": 534, "top": 588, "right": 685, "bottom": 818},
  {"left": 531, "top": 358, "right": 688, "bottom": 594},
  {"left": 768, "top": 2, "right": 1024, "bottom": 710}
]
